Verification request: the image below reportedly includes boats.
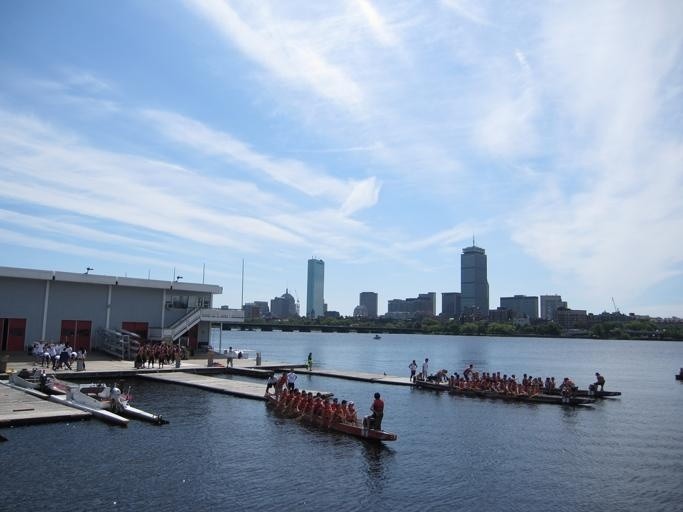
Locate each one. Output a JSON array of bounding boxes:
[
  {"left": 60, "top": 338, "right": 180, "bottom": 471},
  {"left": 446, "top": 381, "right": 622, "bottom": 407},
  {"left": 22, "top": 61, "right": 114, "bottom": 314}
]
[
  {"left": 263, "top": 388, "right": 398, "bottom": 443},
  {"left": 13, "top": 369, "right": 167, "bottom": 430},
  {"left": 414, "top": 365, "right": 621, "bottom": 406}
]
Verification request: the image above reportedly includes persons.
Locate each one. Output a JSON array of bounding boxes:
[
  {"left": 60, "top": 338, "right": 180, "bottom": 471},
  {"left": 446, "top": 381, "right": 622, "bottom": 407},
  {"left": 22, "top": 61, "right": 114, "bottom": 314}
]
[
  {"left": 416, "top": 358, "right": 579, "bottom": 405},
  {"left": 680, "top": 368, "right": 683, "bottom": 376},
  {"left": 594, "top": 373, "right": 605, "bottom": 392},
  {"left": 307, "top": 353, "right": 313, "bottom": 371},
  {"left": 18, "top": 369, "right": 29, "bottom": 379},
  {"left": 39, "top": 370, "right": 47, "bottom": 390},
  {"left": 369, "top": 393, "right": 384, "bottom": 431},
  {"left": 265, "top": 367, "right": 357, "bottom": 429},
  {"left": 133, "top": 344, "right": 184, "bottom": 369},
  {"left": 32, "top": 342, "right": 87, "bottom": 375},
  {"left": 408, "top": 360, "right": 417, "bottom": 381},
  {"left": 226, "top": 347, "right": 234, "bottom": 368},
  {"left": 45, "top": 375, "right": 55, "bottom": 389}
]
